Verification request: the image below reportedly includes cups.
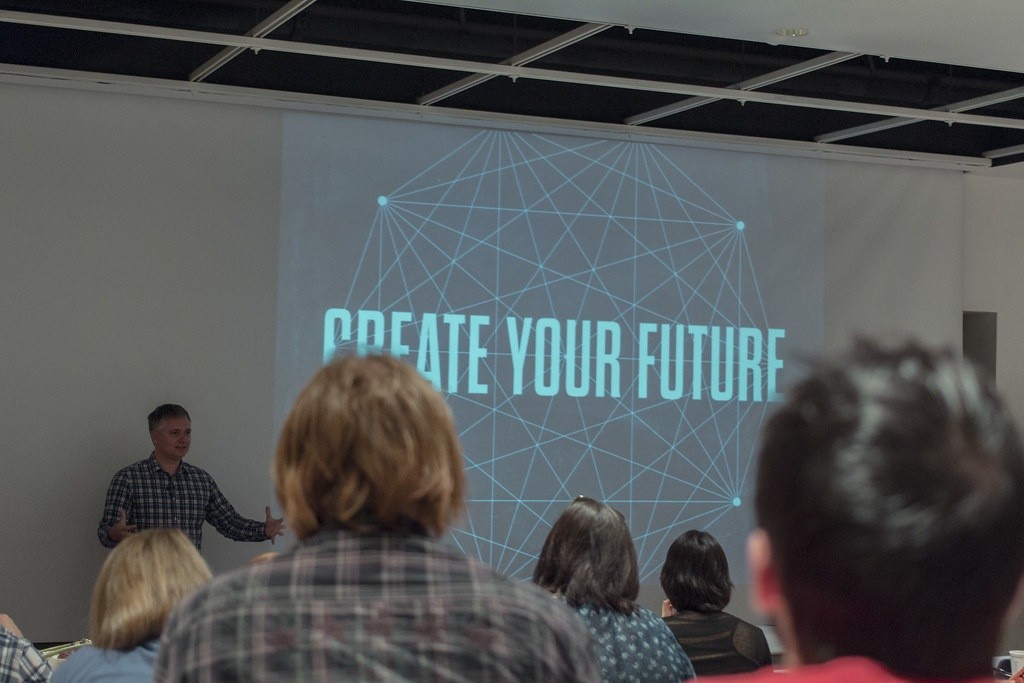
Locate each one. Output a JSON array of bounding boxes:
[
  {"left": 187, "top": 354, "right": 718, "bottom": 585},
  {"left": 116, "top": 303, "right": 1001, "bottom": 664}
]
[{"left": 1009, "top": 650, "right": 1024, "bottom": 675}]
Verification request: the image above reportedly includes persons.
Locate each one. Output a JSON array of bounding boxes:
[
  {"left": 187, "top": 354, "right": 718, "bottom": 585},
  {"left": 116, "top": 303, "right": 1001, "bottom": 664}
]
[
  {"left": 97, "top": 404, "right": 286, "bottom": 555},
  {"left": 0, "top": 614, "right": 53, "bottom": 683},
  {"left": 532, "top": 496, "right": 696, "bottom": 683},
  {"left": 661, "top": 530, "right": 773, "bottom": 677},
  {"left": 155, "top": 356, "right": 601, "bottom": 683},
  {"left": 691, "top": 338, "right": 1024, "bottom": 683},
  {"left": 49, "top": 529, "right": 212, "bottom": 683}
]
